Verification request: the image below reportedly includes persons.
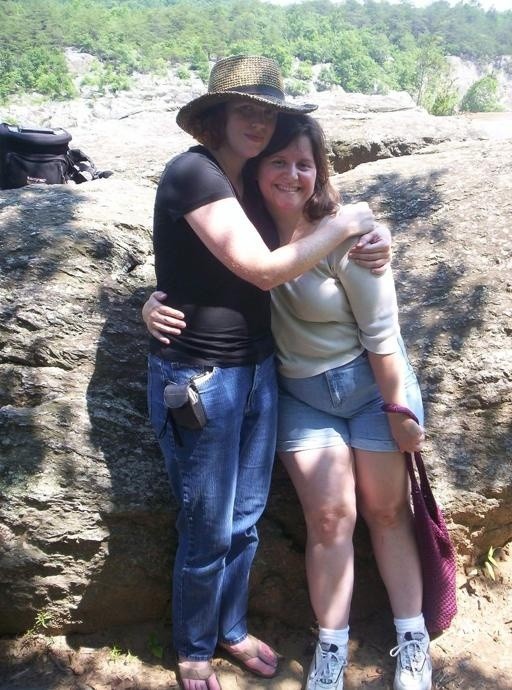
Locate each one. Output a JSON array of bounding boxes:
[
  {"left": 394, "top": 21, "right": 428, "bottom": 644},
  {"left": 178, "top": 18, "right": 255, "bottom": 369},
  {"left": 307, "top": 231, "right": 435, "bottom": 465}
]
[
  {"left": 140, "top": 111, "right": 435, "bottom": 690},
  {"left": 140, "top": 54, "right": 394, "bottom": 690}
]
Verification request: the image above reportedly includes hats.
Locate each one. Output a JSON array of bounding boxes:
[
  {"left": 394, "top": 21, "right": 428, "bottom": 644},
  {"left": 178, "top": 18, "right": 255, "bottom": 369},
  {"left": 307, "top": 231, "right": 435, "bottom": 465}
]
[{"left": 176, "top": 55, "right": 318, "bottom": 143}]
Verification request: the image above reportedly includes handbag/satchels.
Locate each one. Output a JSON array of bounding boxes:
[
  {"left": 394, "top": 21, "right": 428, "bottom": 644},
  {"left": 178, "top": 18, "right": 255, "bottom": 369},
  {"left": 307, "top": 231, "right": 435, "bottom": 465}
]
[
  {"left": 0, "top": 123, "right": 72, "bottom": 190},
  {"left": 411, "top": 482, "right": 457, "bottom": 633}
]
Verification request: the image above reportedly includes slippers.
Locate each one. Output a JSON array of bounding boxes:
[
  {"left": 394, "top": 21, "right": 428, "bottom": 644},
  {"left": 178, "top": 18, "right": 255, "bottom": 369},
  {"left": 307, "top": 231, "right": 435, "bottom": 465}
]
[
  {"left": 175, "top": 652, "right": 222, "bottom": 690},
  {"left": 217, "top": 633, "right": 279, "bottom": 678}
]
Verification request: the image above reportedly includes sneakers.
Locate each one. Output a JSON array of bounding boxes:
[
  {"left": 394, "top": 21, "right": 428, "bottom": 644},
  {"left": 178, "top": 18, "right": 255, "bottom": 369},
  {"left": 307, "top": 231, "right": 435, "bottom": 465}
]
[
  {"left": 390, "top": 626, "right": 432, "bottom": 690},
  {"left": 305, "top": 640, "right": 349, "bottom": 690}
]
